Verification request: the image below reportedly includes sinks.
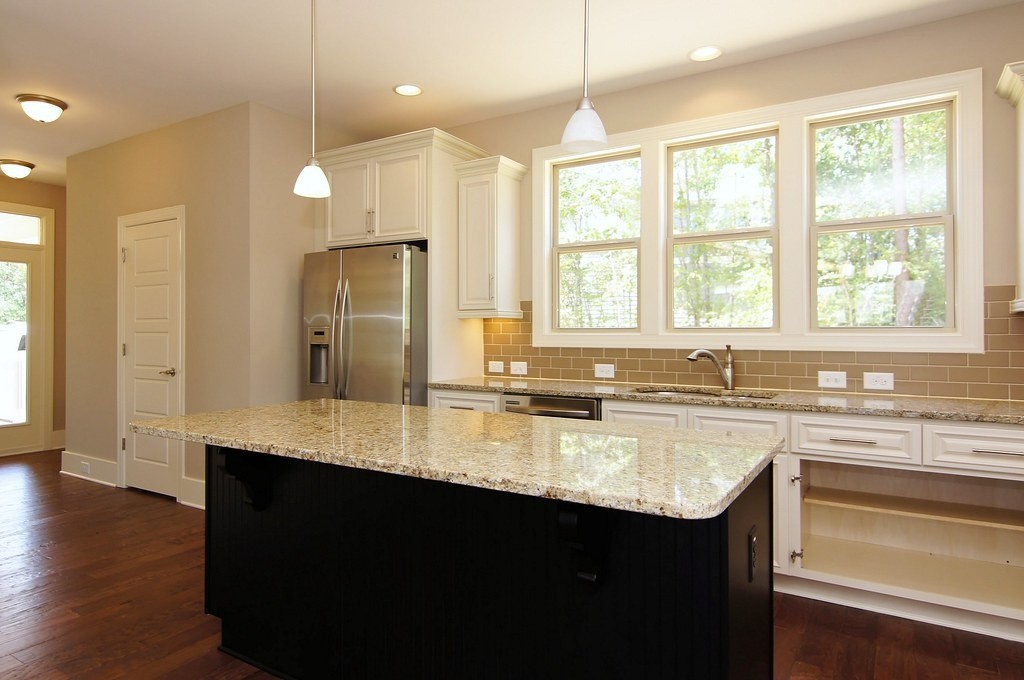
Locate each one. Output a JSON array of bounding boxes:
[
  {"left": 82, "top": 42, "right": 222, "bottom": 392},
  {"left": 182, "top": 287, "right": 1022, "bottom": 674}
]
[{"left": 629, "top": 387, "right": 778, "bottom": 401}]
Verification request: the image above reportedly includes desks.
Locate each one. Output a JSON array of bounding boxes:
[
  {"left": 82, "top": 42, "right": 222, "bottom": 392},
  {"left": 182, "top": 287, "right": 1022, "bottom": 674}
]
[{"left": 130, "top": 398, "right": 776, "bottom": 680}]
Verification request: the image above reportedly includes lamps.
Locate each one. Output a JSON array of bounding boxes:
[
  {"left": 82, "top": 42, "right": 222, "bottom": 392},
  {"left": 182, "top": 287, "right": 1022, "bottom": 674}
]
[
  {"left": 0, "top": 159, "right": 35, "bottom": 180},
  {"left": 560, "top": 0, "right": 609, "bottom": 151},
  {"left": 16, "top": 94, "right": 68, "bottom": 124},
  {"left": 294, "top": 1, "right": 331, "bottom": 199}
]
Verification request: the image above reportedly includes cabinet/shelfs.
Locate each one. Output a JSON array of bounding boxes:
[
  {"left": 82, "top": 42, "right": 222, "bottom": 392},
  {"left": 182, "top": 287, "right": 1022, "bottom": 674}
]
[
  {"left": 424, "top": 389, "right": 500, "bottom": 414},
  {"left": 323, "top": 145, "right": 428, "bottom": 249},
  {"left": 457, "top": 170, "right": 522, "bottom": 319},
  {"left": 792, "top": 456, "right": 1024, "bottom": 633},
  {"left": 792, "top": 412, "right": 1024, "bottom": 618},
  {"left": 600, "top": 400, "right": 790, "bottom": 576}
]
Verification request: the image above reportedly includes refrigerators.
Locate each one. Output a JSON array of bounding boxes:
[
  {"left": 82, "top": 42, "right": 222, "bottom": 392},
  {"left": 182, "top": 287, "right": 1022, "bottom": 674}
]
[{"left": 298, "top": 244, "right": 427, "bottom": 407}]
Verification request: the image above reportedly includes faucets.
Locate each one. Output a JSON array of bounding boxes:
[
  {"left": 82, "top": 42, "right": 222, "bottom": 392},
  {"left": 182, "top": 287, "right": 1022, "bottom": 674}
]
[{"left": 685, "top": 344, "right": 733, "bottom": 389}]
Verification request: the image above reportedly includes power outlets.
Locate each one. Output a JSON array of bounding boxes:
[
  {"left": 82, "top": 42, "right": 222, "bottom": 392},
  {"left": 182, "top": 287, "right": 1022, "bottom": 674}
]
[{"left": 863, "top": 372, "right": 894, "bottom": 390}]
